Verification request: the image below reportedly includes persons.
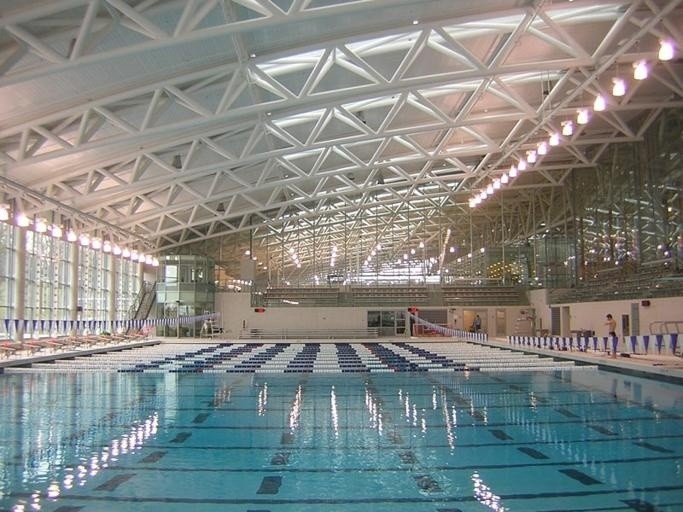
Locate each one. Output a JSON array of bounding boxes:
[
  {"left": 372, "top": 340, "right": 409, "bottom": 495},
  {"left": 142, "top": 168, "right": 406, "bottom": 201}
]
[
  {"left": 473, "top": 313, "right": 482, "bottom": 332},
  {"left": 604, "top": 313, "right": 617, "bottom": 337}
]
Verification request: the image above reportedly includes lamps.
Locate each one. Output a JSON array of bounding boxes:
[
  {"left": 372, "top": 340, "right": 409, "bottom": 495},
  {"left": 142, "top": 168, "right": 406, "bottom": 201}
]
[
  {"left": 0, "top": 176, "right": 160, "bottom": 266},
  {"left": 467, "top": 1, "right": 683, "bottom": 208}
]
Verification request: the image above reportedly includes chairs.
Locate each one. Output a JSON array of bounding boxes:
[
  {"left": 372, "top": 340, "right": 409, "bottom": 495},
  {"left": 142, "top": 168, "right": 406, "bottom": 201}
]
[{"left": 0, "top": 334, "right": 154, "bottom": 359}]
